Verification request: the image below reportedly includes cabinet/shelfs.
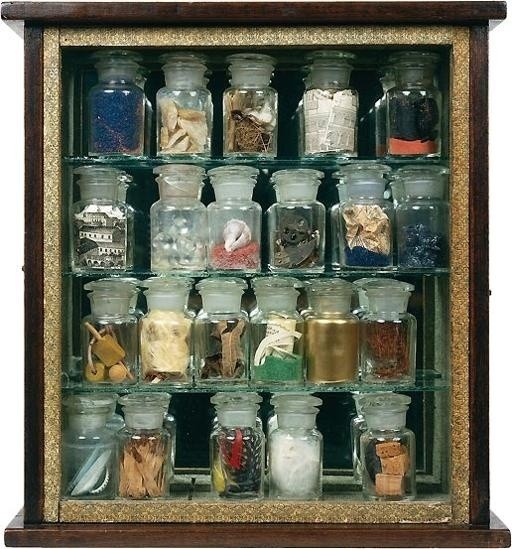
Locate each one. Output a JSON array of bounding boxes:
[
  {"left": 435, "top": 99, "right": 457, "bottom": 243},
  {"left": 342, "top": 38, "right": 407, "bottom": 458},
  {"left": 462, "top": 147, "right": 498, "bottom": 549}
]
[{"left": 3, "top": 1, "right": 512, "bottom": 548}]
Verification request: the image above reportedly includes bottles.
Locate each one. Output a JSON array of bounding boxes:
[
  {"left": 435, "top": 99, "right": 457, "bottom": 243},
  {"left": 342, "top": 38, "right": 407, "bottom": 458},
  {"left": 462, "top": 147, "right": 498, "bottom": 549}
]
[
  {"left": 164, "top": 397, "right": 176, "bottom": 478},
  {"left": 59, "top": 396, "right": 119, "bottom": 501},
  {"left": 116, "top": 174, "right": 137, "bottom": 271},
  {"left": 397, "top": 165, "right": 451, "bottom": 268},
  {"left": 264, "top": 414, "right": 277, "bottom": 466},
  {"left": 129, "top": 282, "right": 145, "bottom": 319},
  {"left": 249, "top": 277, "right": 304, "bottom": 386},
  {"left": 139, "top": 279, "right": 192, "bottom": 388},
  {"left": 296, "top": 65, "right": 316, "bottom": 157},
  {"left": 330, "top": 172, "right": 347, "bottom": 269},
  {"left": 374, "top": 66, "right": 398, "bottom": 157},
  {"left": 337, "top": 162, "right": 394, "bottom": 270},
  {"left": 360, "top": 392, "right": 415, "bottom": 500},
  {"left": 388, "top": 171, "right": 407, "bottom": 211},
  {"left": 150, "top": 166, "right": 208, "bottom": 271},
  {"left": 119, "top": 391, "right": 172, "bottom": 499},
  {"left": 352, "top": 278, "right": 370, "bottom": 317},
  {"left": 222, "top": 53, "right": 280, "bottom": 158},
  {"left": 80, "top": 280, "right": 138, "bottom": 382},
  {"left": 70, "top": 166, "right": 128, "bottom": 272},
  {"left": 209, "top": 391, "right": 265, "bottom": 503},
  {"left": 108, "top": 394, "right": 126, "bottom": 432},
  {"left": 268, "top": 393, "right": 324, "bottom": 502},
  {"left": 132, "top": 64, "right": 153, "bottom": 157},
  {"left": 268, "top": 169, "right": 327, "bottom": 272},
  {"left": 302, "top": 59, "right": 360, "bottom": 158},
  {"left": 155, "top": 59, "right": 213, "bottom": 160},
  {"left": 359, "top": 282, "right": 416, "bottom": 386},
  {"left": 192, "top": 280, "right": 249, "bottom": 386},
  {"left": 305, "top": 279, "right": 360, "bottom": 386},
  {"left": 299, "top": 278, "right": 322, "bottom": 319},
  {"left": 385, "top": 51, "right": 442, "bottom": 156},
  {"left": 86, "top": 50, "right": 145, "bottom": 157},
  {"left": 206, "top": 163, "right": 262, "bottom": 270},
  {"left": 349, "top": 392, "right": 367, "bottom": 479}
]
